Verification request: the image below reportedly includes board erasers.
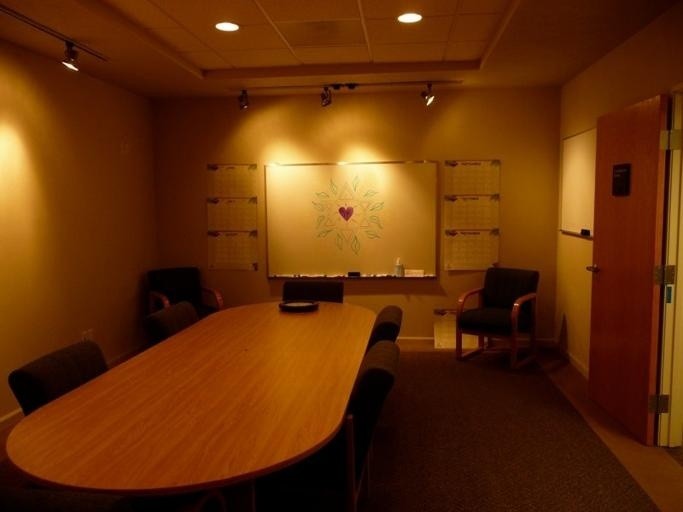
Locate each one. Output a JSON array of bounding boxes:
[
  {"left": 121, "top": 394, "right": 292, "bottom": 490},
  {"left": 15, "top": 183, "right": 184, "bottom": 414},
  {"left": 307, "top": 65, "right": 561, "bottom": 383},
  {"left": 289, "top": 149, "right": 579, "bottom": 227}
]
[
  {"left": 582, "top": 230, "right": 590, "bottom": 236},
  {"left": 348, "top": 272, "right": 360, "bottom": 276}
]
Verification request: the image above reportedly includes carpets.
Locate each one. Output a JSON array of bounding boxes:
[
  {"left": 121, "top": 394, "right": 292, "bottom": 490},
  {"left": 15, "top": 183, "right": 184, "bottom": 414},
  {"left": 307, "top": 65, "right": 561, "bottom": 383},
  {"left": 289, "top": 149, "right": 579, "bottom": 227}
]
[{"left": 4, "top": 348, "right": 664, "bottom": 512}]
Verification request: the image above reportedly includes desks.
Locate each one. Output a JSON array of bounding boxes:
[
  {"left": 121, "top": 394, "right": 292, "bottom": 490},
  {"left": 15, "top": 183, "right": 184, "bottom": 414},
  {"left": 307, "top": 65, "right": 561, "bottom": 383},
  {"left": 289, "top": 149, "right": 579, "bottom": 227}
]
[{"left": 4, "top": 299, "right": 377, "bottom": 508}]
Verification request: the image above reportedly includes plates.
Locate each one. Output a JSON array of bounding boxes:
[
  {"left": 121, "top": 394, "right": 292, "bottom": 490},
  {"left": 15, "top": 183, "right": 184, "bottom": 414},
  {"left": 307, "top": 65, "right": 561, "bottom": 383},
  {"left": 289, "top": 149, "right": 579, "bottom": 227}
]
[{"left": 278, "top": 300, "right": 319, "bottom": 308}]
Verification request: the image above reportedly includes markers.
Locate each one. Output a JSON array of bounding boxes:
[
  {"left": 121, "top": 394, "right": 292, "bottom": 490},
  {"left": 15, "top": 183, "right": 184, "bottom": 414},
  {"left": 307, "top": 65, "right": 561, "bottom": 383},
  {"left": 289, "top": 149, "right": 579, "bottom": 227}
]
[{"left": 274, "top": 274, "right": 396, "bottom": 278}]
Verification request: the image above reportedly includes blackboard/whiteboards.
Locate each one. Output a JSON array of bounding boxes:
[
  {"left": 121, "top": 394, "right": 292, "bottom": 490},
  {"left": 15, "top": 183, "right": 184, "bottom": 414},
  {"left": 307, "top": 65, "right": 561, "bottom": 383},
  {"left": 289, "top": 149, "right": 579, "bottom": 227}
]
[
  {"left": 263, "top": 160, "right": 440, "bottom": 280},
  {"left": 559, "top": 126, "right": 597, "bottom": 239}
]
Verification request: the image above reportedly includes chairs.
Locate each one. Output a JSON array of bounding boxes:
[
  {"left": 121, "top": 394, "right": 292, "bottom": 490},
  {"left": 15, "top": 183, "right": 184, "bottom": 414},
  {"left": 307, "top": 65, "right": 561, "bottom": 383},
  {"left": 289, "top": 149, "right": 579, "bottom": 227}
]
[
  {"left": 4, "top": 264, "right": 224, "bottom": 419},
  {"left": 453, "top": 265, "right": 541, "bottom": 370},
  {"left": 247, "top": 305, "right": 409, "bottom": 511},
  {"left": 280, "top": 279, "right": 343, "bottom": 304}
]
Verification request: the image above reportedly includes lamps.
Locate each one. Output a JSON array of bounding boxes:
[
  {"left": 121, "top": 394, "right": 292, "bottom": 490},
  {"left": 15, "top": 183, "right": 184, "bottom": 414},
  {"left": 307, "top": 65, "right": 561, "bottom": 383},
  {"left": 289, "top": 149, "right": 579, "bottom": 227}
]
[
  {"left": 320, "top": 86, "right": 332, "bottom": 106},
  {"left": 419, "top": 84, "right": 435, "bottom": 107},
  {"left": 60, "top": 42, "right": 80, "bottom": 73},
  {"left": 237, "top": 90, "right": 250, "bottom": 111}
]
